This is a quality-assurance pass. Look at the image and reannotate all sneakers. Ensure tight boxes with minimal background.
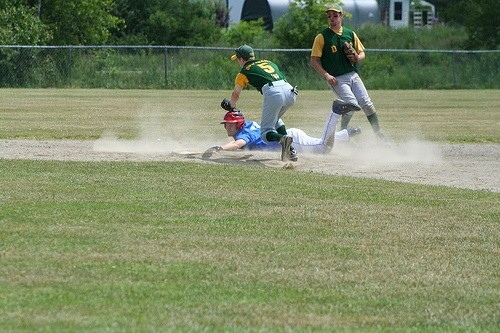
[347,126,361,137]
[278,135,298,162]
[331,99,361,114]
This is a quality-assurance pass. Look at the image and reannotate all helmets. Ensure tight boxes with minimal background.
[220,111,245,128]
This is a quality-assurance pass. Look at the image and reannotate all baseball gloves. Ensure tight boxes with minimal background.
[343,42,359,64]
[220,98,236,112]
[202,145,223,160]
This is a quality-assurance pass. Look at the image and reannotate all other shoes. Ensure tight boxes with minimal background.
[374,132,384,139]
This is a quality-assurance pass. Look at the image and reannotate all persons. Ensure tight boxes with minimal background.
[220,44,299,161]
[202,99,361,159]
[309,2,385,142]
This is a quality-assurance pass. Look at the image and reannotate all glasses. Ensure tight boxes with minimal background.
[327,15,340,18]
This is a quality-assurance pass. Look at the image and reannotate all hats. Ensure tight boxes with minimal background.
[231,44,254,61]
[324,3,343,14]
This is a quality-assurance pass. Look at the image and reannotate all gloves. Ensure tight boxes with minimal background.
[221,98,236,111]
[202,146,223,160]
